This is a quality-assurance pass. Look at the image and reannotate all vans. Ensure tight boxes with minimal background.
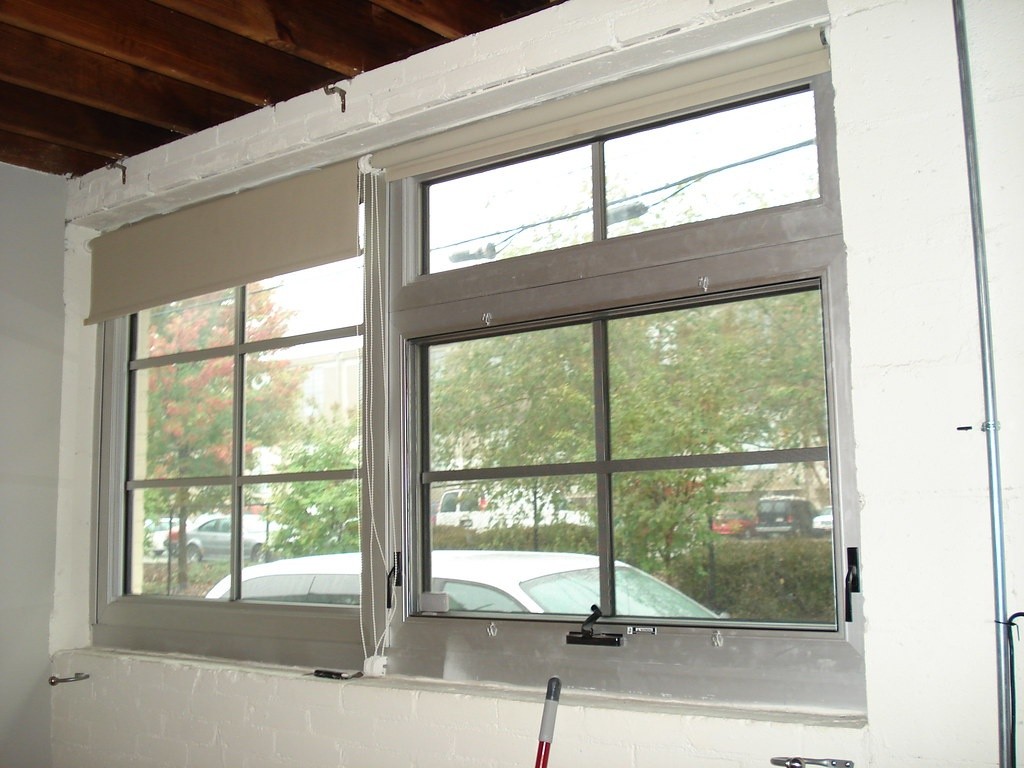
[435,489,550,530]
[205,549,728,618]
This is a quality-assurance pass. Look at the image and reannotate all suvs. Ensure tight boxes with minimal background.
[753,495,819,538]
[145,517,191,557]
[813,506,834,537]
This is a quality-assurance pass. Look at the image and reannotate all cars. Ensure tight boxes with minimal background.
[165,515,303,562]
[713,512,754,538]
